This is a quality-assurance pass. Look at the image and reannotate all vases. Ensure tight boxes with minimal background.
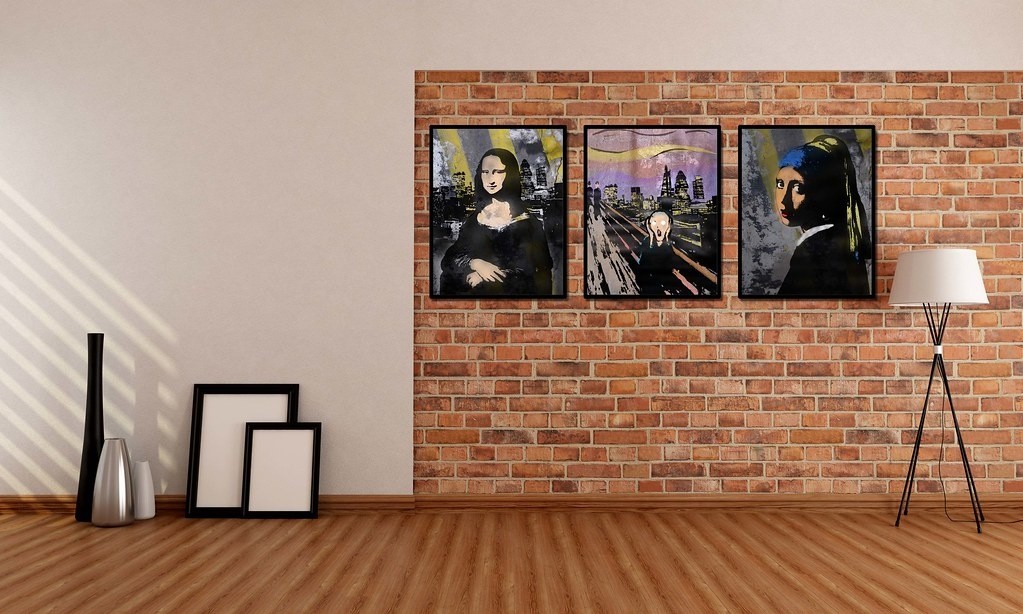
[134,460,155,519]
[92,438,134,527]
[75,333,111,522]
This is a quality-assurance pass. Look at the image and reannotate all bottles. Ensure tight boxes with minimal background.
[92,438,157,527]
[74,332,108,521]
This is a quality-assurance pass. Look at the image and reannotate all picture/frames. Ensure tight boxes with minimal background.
[241,422,322,518]
[185,384,299,518]
[738,125,875,299]
[428,125,567,299]
[584,125,725,300]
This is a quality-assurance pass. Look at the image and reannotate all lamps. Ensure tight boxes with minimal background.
[888,248,990,533]
